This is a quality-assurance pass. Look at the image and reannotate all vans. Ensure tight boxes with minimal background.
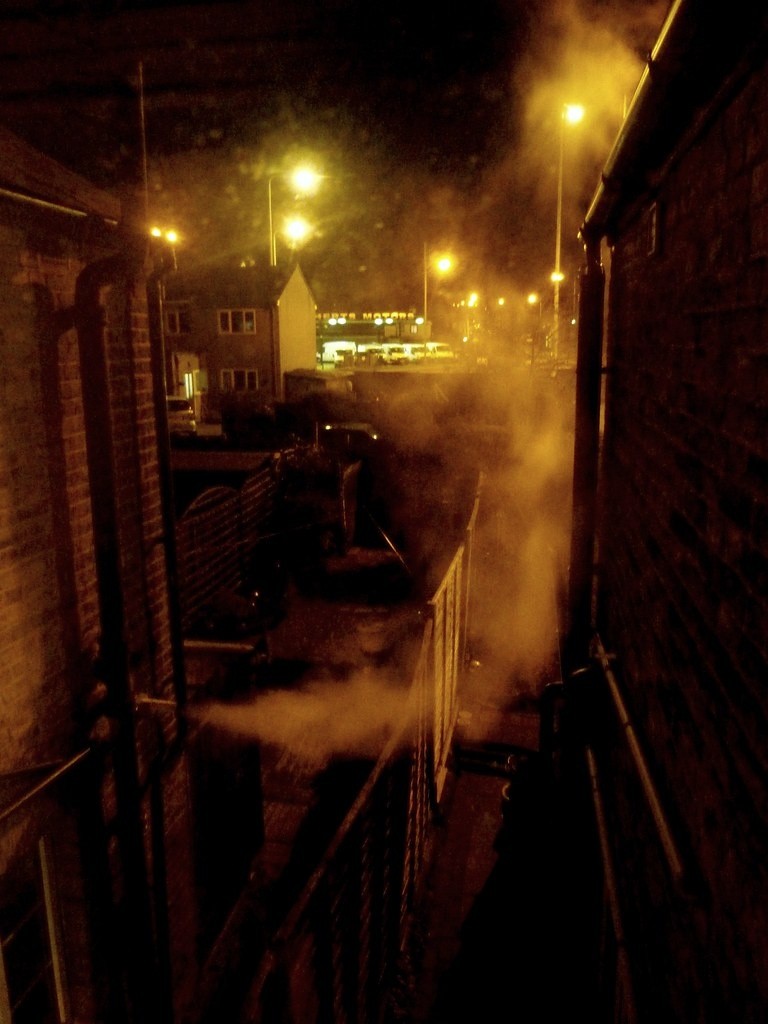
[332,342,455,369]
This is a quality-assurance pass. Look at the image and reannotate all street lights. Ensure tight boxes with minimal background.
[268,164,319,267]
[549,100,587,380]
